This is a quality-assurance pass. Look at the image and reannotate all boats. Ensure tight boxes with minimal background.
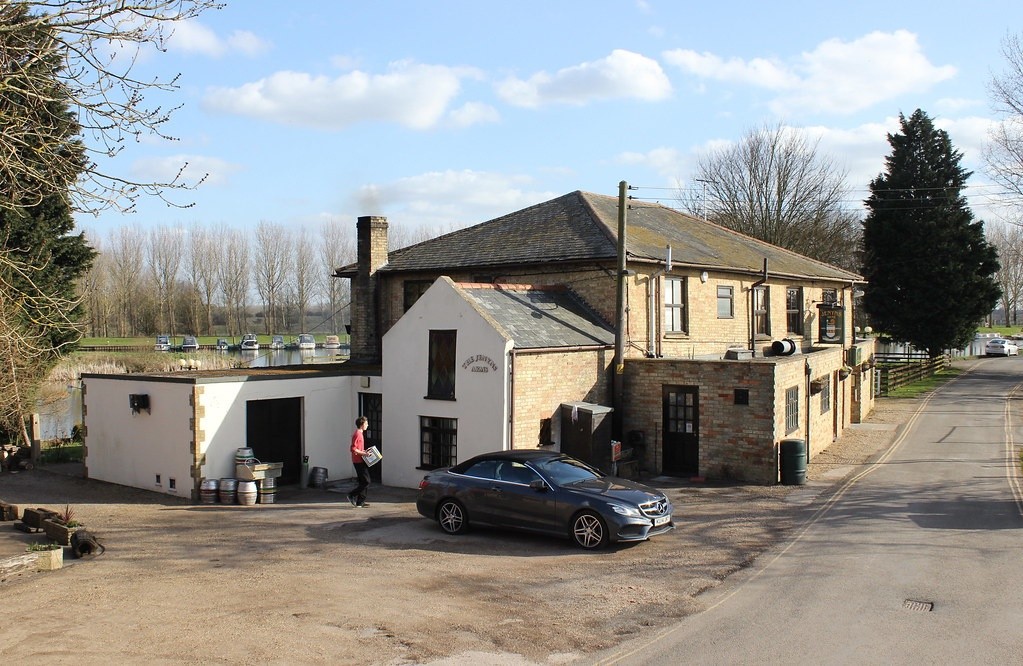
[240,334,260,351]
[296,335,316,349]
[181,337,199,351]
[154,334,171,352]
[323,335,341,349]
[269,336,286,350]
[217,337,229,350]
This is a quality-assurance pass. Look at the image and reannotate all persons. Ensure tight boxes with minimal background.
[347,417,370,508]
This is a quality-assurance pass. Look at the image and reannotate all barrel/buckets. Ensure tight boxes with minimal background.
[237,481,257,505]
[779,439,806,485]
[235,447,255,464]
[219,478,238,504]
[310,467,328,488]
[259,478,274,503]
[200,479,219,503]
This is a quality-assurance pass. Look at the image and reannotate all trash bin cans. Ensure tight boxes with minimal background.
[781,440,807,485]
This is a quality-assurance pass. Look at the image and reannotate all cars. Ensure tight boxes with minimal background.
[417,448,676,551]
[985,339,1020,356]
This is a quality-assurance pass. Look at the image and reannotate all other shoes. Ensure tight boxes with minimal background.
[356,502,370,508]
[346,494,356,507]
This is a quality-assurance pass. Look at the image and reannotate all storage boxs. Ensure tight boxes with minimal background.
[361,446,382,468]
[610,441,622,461]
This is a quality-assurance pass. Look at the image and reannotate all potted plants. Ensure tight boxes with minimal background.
[812,375,826,393]
[862,359,871,370]
[43,504,86,546]
[839,363,850,380]
